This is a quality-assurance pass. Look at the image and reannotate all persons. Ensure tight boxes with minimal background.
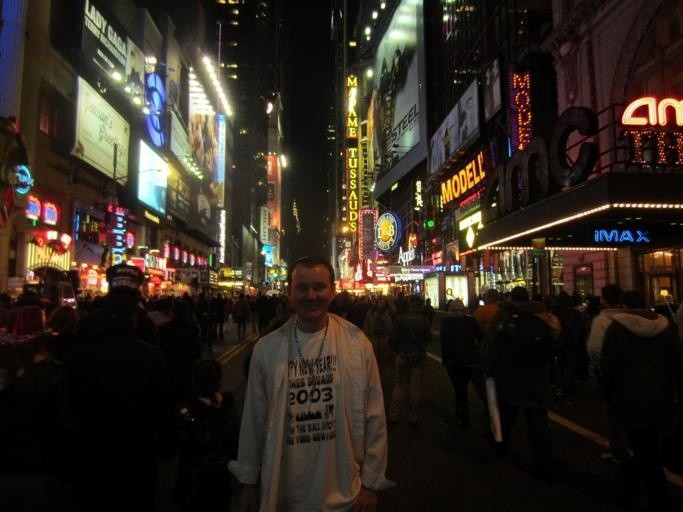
[0,257,683,512]
